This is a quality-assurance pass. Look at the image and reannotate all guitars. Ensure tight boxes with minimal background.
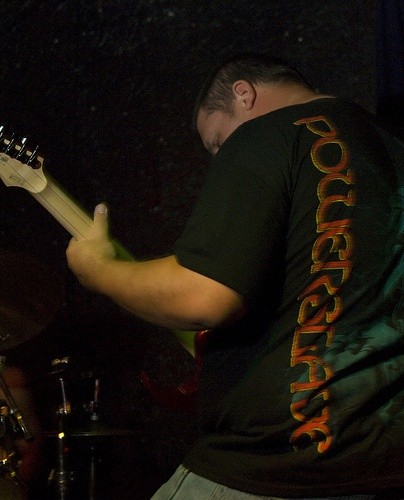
[1,126,256,425]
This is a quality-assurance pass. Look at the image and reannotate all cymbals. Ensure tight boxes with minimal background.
[72,426,147,436]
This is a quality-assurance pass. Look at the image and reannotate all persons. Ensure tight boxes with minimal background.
[64,50,404,500]
